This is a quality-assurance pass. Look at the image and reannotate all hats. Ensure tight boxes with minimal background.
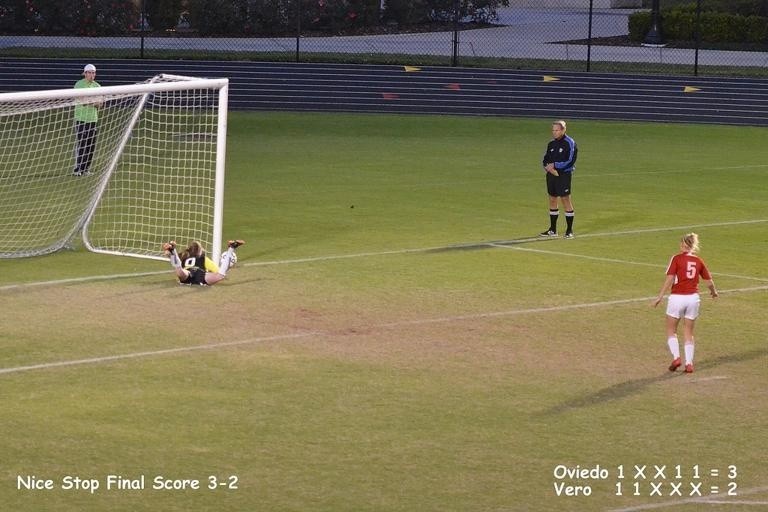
[84,64,96,72]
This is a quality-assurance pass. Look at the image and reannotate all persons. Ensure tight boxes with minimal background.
[649,233,718,373]
[71,63,104,176]
[539,120,577,239]
[162,238,244,286]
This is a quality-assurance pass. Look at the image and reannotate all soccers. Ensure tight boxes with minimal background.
[220,252,236,267]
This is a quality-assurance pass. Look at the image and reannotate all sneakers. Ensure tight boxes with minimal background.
[541,227,575,240]
[163,241,176,250]
[228,240,244,247]
[669,357,694,373]
